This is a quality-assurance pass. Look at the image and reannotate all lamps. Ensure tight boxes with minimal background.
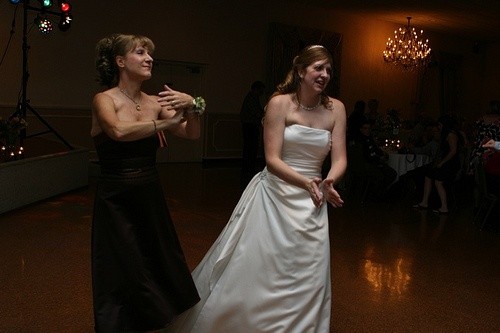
[8,0,73,34]
[383,16,431,73]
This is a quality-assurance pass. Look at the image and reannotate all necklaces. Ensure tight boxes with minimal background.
[295,96,322,111]
[118,87,142,111]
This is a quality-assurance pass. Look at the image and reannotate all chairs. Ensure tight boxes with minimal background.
[471,157,500,231]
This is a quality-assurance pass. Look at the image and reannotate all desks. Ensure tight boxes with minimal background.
[386,154,432,184]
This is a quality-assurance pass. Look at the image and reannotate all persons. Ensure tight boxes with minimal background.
[167,44,347,333]
[325,99,500,214]
[89,34,206,333]
[240,81,264,177]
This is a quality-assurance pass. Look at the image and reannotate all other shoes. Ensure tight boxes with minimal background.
[432,209,448,214]
[412,204,428,208]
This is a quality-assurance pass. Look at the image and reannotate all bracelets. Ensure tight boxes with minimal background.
[192,96,206,119]
[152,120,157,135]
